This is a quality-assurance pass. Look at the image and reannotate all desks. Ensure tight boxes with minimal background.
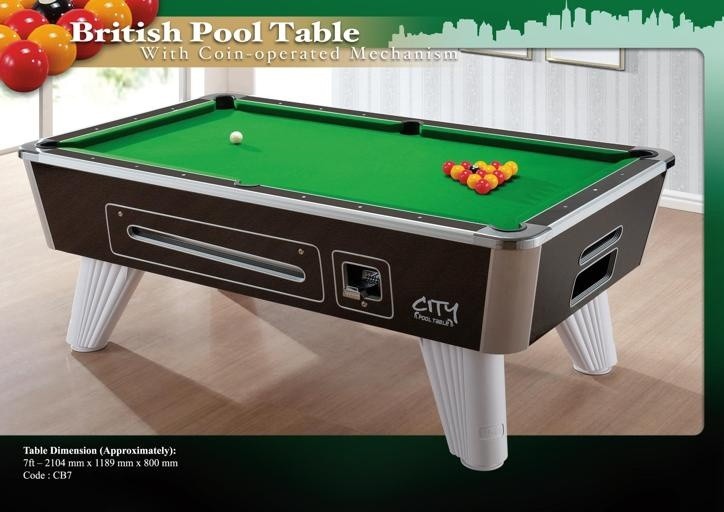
[13,88,677,474]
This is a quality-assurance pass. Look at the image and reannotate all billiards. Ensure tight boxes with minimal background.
[230,130,243,144]
[444,160,518,195]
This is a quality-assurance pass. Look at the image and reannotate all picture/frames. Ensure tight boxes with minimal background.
[543,47,626,73]
[457,47,533,62]
